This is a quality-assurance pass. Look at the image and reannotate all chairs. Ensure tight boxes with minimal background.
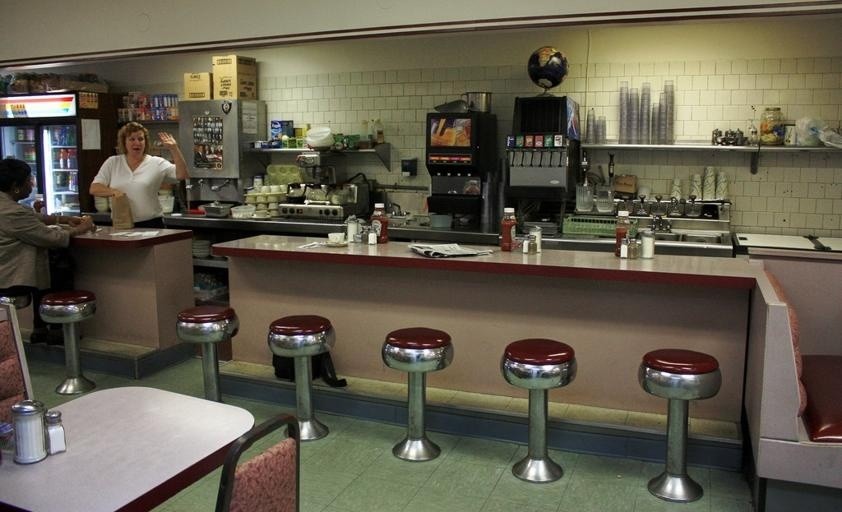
[0,297,43,445]
[214,410,302,511]
[741,265,840,512]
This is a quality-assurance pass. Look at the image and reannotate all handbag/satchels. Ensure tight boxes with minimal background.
[272,353,346,387]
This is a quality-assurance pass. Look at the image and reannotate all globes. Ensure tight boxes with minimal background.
[526,46,571,98]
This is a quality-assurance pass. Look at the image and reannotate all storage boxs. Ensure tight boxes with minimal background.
[183,71,215,103]
[269,119,294,148]
[210,51,260,103]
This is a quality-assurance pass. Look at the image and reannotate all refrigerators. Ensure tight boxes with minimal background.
[1,89,125,215]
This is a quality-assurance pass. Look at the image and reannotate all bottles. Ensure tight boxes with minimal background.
[523,254,541,265]
[254,176,263,191]
[50,126,78,208]
[711,128,743,147]
[348,202,388,245]
[759,106,785,145]
[783,123,796,146]
[639,229,655,259]
[748,127,757,144]
[522,226,542,254]
[614,210,638,259]
[366,118,384,149]
[500,206,517,252]
[12,398,67,465]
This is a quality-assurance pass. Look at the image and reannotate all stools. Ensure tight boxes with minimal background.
[379,325,457,462]
[2,293,33,357]
[36,289,103,398]
[265,314,338,443]
[631,344,723,504]
[496,336,578,484]
[173,303,243,406]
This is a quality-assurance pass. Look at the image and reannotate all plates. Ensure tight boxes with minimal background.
[326,241,348,247]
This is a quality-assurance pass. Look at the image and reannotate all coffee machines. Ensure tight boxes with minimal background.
[177,99,268,211]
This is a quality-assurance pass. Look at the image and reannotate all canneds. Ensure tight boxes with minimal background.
[17,127,36,142]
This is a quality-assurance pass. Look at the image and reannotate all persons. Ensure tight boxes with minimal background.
[89,121,190,228]
[0,159,93,349]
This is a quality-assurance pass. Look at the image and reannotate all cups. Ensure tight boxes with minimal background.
[327,232,346,242]
[669,177,682,199]
[689,164,730,202]
[575,182,594,211]
[461,91,492,113]
[244,184,287,218]
[594,183,614,213]
[158,195,175,214]
[618,78,675,146]
[585,109,606,145]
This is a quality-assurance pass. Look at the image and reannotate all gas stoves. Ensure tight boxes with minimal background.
[278,202,371,220]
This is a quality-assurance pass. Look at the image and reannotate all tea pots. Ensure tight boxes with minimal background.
[285,181,354,205]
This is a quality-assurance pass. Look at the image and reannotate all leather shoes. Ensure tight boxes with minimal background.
[31,333,63,345]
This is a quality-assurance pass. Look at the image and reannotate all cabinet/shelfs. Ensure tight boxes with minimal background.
[115,119,180,152]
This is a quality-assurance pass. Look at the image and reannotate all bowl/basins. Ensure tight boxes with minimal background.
[231,204,256,217]
[93,194,112,213]
[432,213,452,228]
[192,238,226,260]
